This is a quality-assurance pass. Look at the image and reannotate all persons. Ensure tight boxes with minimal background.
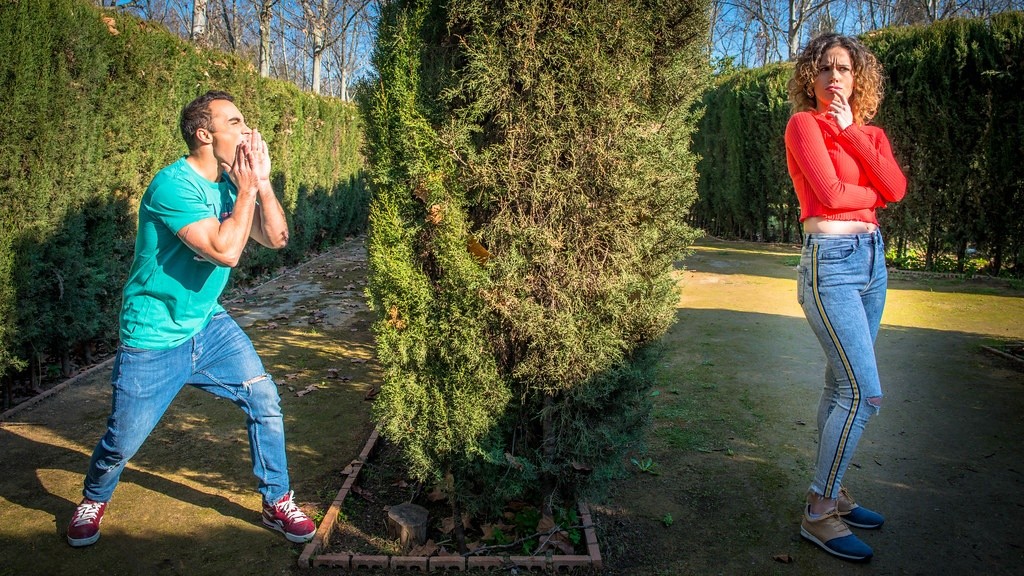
[785,34,907,559]
[67,90,316,546]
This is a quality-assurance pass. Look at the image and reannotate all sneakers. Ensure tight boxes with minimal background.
[805,486,885,529]
[799,503,872,559]
[262,491,317,542]
[67,498,107,546]
